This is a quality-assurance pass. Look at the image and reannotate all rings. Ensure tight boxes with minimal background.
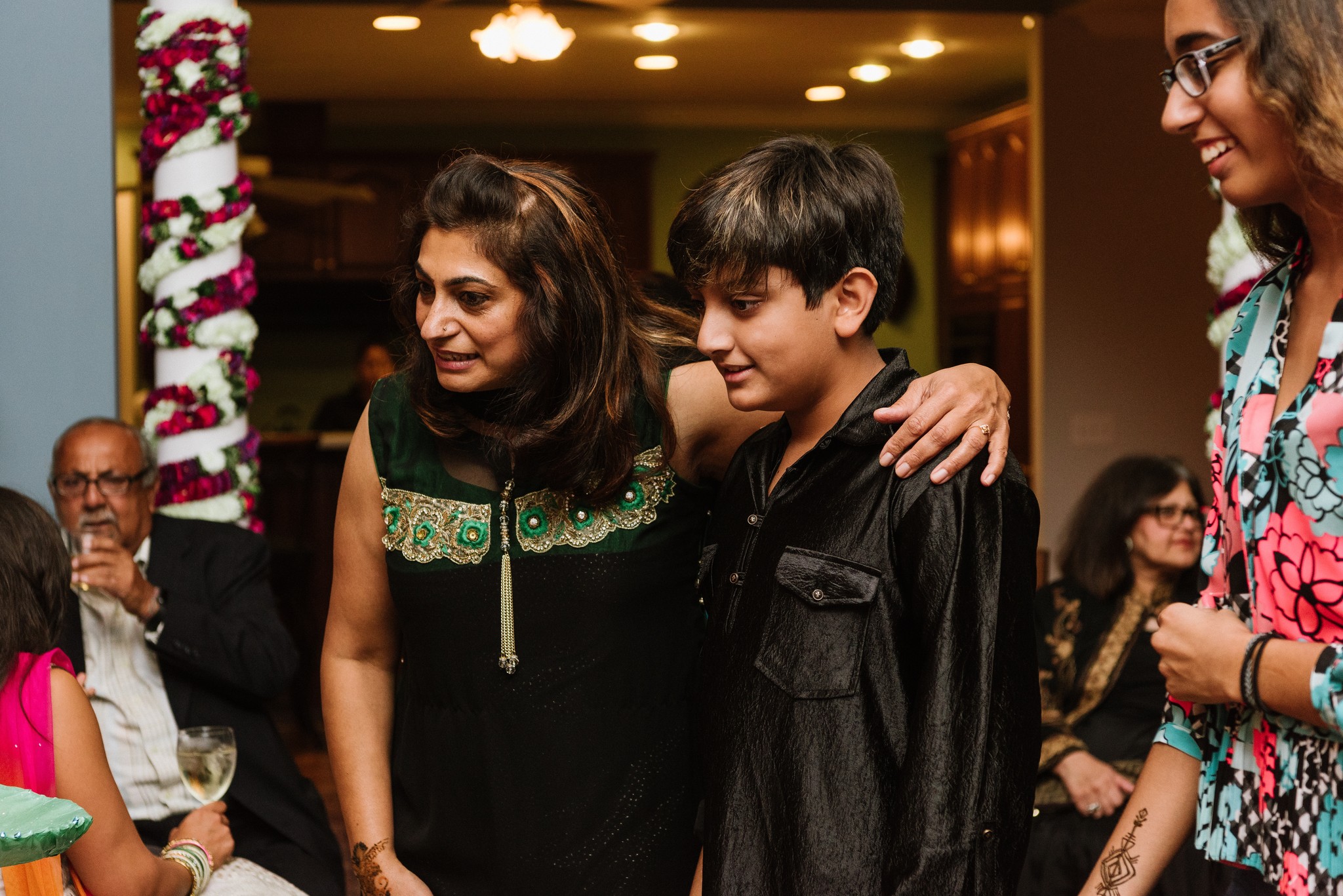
[969,424,990,440]
[1087,803,1099,815]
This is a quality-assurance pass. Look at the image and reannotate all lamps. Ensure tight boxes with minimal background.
[470,0,580,63]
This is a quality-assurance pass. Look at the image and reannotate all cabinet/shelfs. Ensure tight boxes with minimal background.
[942,99,1027,487]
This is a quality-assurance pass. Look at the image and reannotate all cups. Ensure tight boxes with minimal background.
[176,726,237,808]
[69,531,119,598]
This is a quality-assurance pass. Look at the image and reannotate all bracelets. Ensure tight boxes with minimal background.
[159,838,214,896]
[1236,631,1285,714]
[145,588,167,633]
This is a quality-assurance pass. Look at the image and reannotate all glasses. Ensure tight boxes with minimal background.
[1143,504,1206,527]
[48,465,151,498]
[1158,34,1244,98]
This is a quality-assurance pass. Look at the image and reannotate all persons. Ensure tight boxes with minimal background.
[322,150,1012,896]
[665,135,1043,894]
[0,485,235,896]
[46,416,346,895]
[309,338,403,432]
[1035,453,1206,896]
[1074,0,1343,896]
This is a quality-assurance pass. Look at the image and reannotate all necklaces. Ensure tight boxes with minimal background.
[488,455,524,674]
